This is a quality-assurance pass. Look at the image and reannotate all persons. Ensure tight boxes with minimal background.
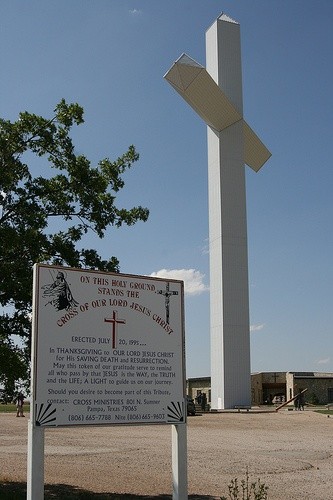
[200,392,207,412]
[297,392,305,410]
[14,392,26,417]
